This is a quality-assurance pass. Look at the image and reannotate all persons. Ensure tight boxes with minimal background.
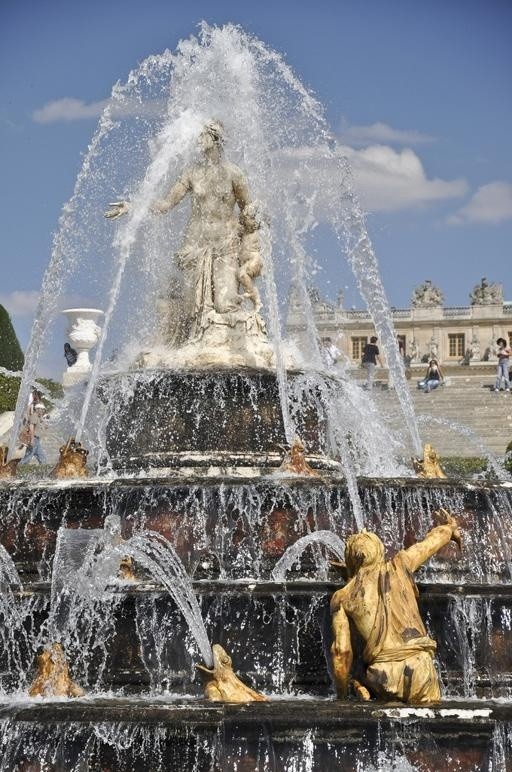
[494,337,512,392]
[17,402,48,467]
[414,279,442,305]
[359,335,385,389]
[418,359,444,392]
[474,277,496,305]
[327,507,465,702]
[237,211,267,314]
[104,118,259,317]
[62,341,77,366]
[426,336,438,358]
[323,337,342,367]
